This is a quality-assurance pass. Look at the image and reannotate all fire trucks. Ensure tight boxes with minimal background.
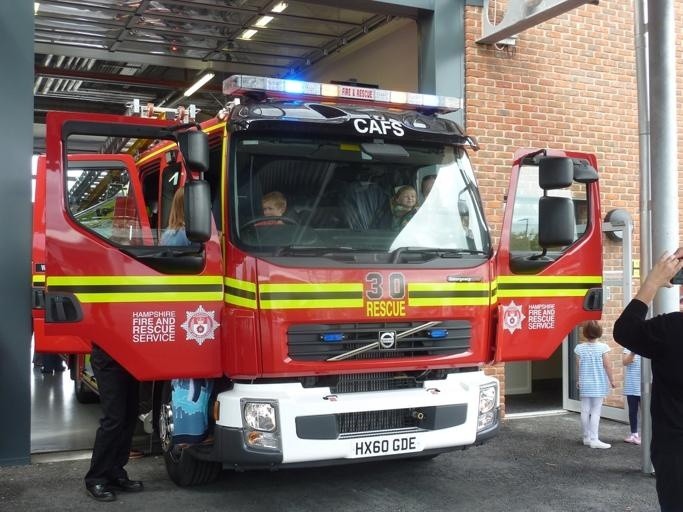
[26,74,607,491]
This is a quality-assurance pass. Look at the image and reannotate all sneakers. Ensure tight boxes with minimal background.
[624,434,635,443]
[590,439,612,450]
[40,366,67,373]
[583,438,591,446]
[633,436,641,446]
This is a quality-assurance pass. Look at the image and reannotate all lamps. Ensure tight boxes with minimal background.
[183,69,216,97]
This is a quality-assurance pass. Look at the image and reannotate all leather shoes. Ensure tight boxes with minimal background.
[86,480,117,502]
[114,477,145,493]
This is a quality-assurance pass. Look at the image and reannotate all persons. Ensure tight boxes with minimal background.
[157,185,213,449]
[40,353,66,373]
[32,350,43,366]
[391,185,418,231]
[254,191,287,226]
[622,347,642,445]
[421,174,438,200]
[82,237,144,503]
[574,320,617,449]
[611,245,683,512]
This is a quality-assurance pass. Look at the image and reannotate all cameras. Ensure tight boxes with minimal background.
[670,267,683,284]
